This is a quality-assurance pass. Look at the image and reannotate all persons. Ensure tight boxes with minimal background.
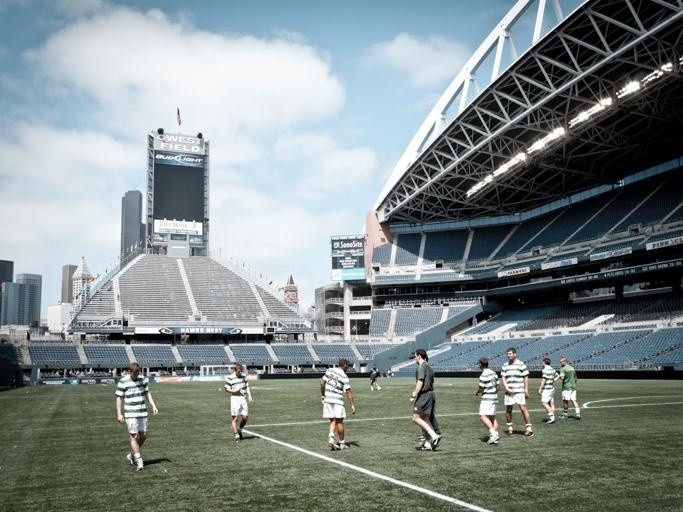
[370,364,382,391]
[538,358,560,424]
[559,358,581,419]
[321,358,356,451]
[387,368,392,378]
[224,364,253,441]
[68,368,94,377]
[409,348,443,451]
[476,358,501,444]
[115,363,158,472]
[501,348,534,436]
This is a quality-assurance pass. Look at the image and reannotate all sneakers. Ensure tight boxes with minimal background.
[370,387,382,391]
[486,433,501,445]
[523,430,534,438]
[431,434,443,451]
[546,412,581,425]
[327,438,349,451]
[133,456,143,472]
[503,428,513,438]
[126,452,135,466]
[233,429,244,441]
[416,440,433,450]
